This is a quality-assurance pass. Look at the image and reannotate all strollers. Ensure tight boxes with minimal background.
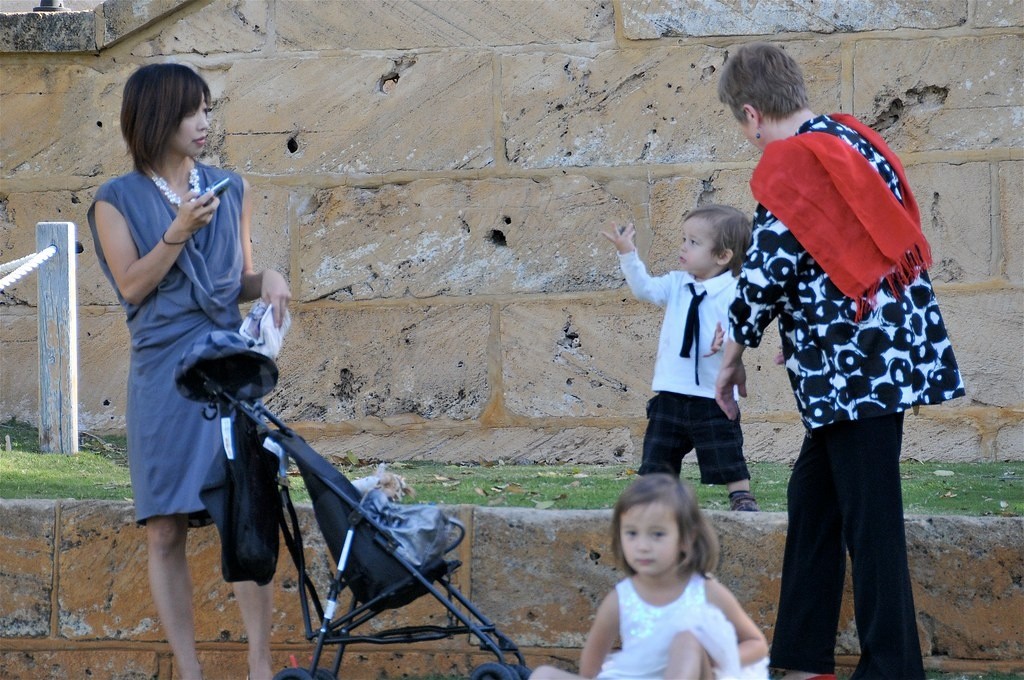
[200,368,532,680]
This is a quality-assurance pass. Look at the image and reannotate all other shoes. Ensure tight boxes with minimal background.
[731,493,758,511]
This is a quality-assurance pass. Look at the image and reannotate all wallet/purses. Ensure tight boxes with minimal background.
[238,302,292,360]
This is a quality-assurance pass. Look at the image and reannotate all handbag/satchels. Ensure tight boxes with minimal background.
[361,486,447,566]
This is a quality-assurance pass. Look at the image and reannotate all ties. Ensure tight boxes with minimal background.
[679,282,710,387]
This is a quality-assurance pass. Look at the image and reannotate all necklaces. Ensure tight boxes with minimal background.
[151,168,201,208]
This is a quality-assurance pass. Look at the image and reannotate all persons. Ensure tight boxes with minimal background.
[526,473,770,680]
[715,44,966,680]
[86,63,292,680]
[601,205,759,512]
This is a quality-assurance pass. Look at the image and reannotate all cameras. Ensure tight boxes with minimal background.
[196,177,230,208]
